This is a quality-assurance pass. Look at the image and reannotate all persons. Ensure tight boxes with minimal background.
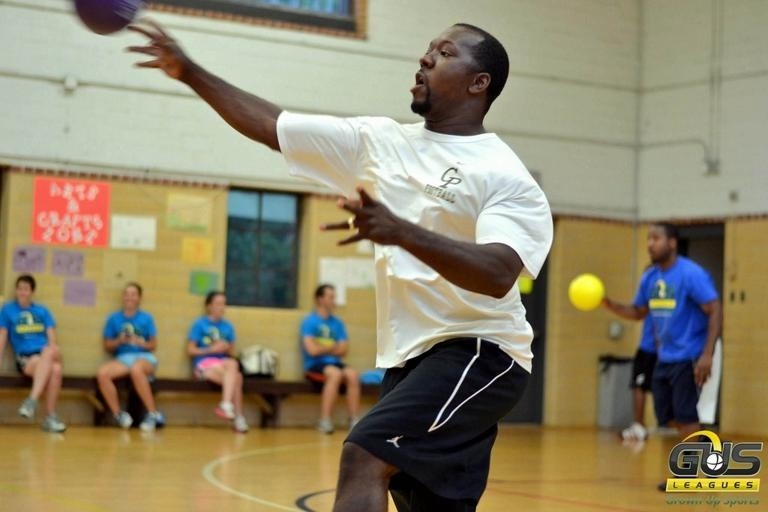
[95,281,168,433]
[185,291,251,433]
[123,16,557,512]
[0,274,68,433]
[298,283,363,434]
[617,316,658,440]
[602,223,724,493]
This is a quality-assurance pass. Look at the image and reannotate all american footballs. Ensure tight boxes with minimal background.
[75,1,136,34]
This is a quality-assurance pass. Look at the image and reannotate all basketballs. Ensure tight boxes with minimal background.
[569,273,604,311]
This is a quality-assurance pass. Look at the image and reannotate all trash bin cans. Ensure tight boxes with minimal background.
[597,354,634,428]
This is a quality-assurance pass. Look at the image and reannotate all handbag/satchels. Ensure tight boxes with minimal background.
[242,344,275,379]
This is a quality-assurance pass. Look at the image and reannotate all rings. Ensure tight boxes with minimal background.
[347,218,356,230]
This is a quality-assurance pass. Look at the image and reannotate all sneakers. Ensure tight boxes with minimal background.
[18,398,66,432]
[115,411,165,430]
[620,422,647,439]
[215,401,248,432]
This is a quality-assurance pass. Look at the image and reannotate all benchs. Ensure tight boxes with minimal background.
[0,377,378,429]
